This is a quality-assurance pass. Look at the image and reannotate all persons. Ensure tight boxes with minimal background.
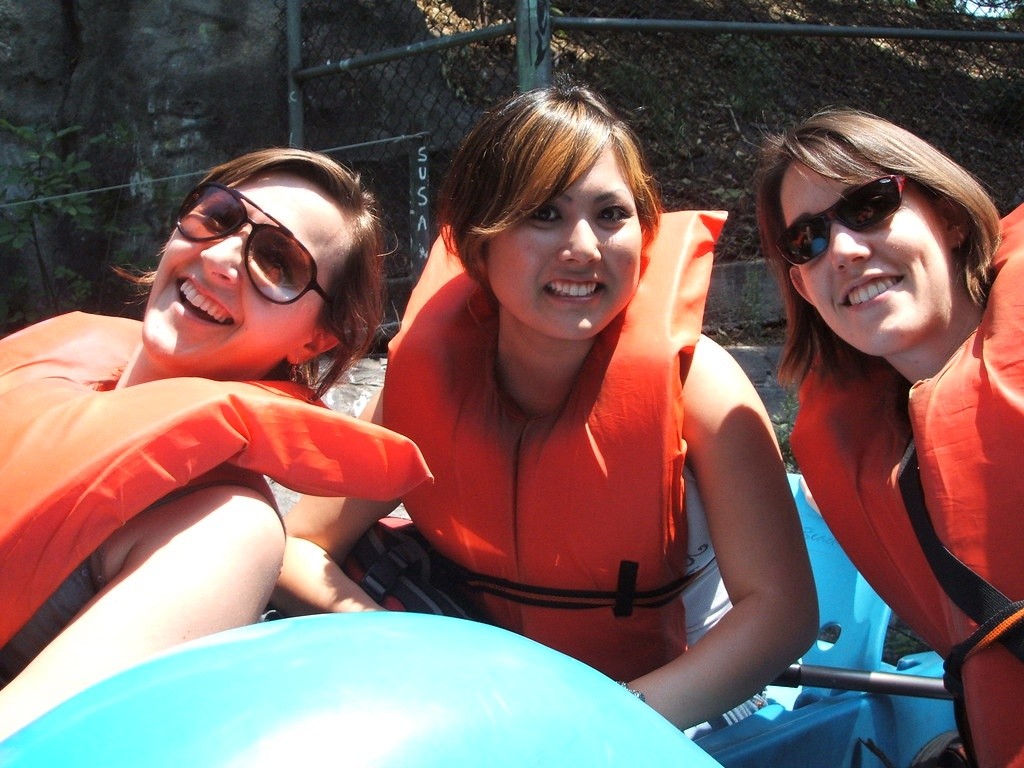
[275,83,820,731]
[755,108,1024,768]
[0,146,436,743]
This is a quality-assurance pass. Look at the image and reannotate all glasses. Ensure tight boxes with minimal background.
[775,175,908,266]
[176,180,338,312]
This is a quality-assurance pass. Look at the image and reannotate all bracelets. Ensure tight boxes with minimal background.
[619,680,646,704]
